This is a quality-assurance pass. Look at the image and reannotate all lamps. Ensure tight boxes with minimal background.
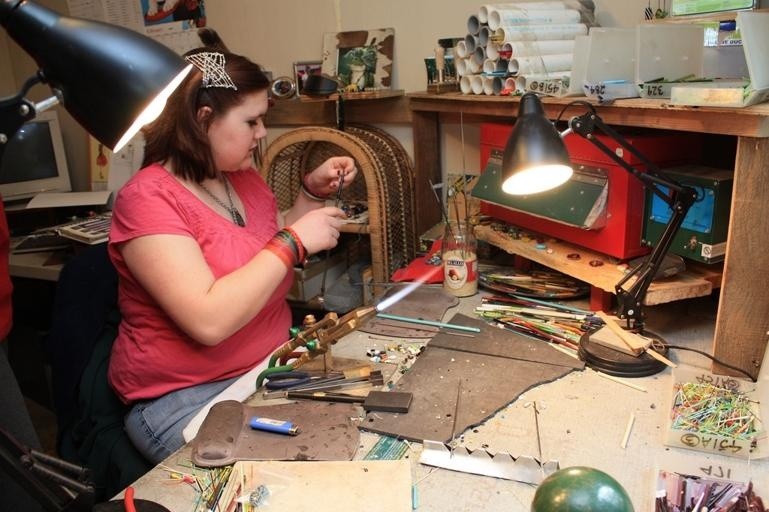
[502,90,701,379]
[1,1,190,154]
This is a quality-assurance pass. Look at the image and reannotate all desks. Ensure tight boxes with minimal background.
[119,263,769,512]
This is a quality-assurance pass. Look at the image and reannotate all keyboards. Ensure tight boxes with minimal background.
[12,234,81,254]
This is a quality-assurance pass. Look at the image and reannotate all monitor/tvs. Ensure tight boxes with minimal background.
[0,109,73,201]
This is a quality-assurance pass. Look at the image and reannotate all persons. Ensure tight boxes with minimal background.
[107,49,359,470]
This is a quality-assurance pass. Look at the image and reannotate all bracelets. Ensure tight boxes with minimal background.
[301,172,331,204]
[263,227,308,270]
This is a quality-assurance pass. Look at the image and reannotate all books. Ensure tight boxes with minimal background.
[61,208,116,246]
[12,226,72,256]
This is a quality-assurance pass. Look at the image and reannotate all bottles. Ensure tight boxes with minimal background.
[441,222,479,298]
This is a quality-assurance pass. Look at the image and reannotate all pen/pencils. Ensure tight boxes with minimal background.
[654,481,742,512]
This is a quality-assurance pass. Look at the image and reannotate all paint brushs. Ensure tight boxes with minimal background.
[473,268,607,351]
